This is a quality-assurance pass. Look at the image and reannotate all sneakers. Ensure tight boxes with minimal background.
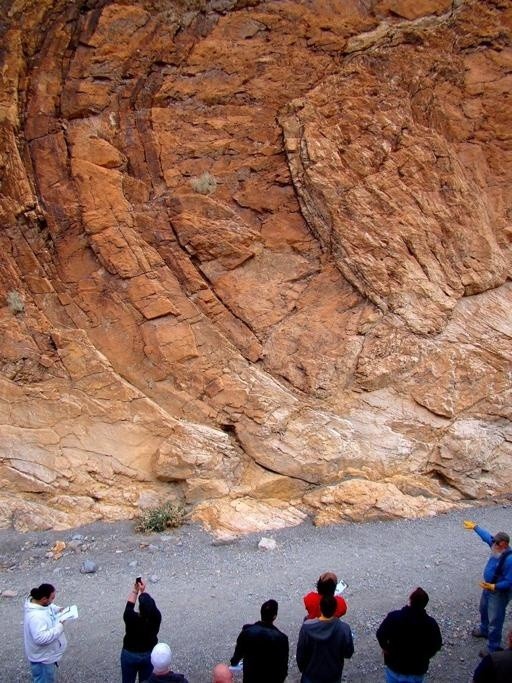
[479,649,488,657]
[473,629,481,636]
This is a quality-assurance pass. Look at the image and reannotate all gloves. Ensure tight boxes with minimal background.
[480,582,496,592]
[463,521,476,529]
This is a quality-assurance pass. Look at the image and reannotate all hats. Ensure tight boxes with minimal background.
[139,593,155,611]
[409,587,429,604]
[151,642,172,672]
[491,532,510,542]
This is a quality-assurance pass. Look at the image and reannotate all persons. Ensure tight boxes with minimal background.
[296,595,354,683]
[120,576,161,683]
[472,627,511,683]
[229,599,289,683]
[375,587,441,683]
[23,583,66,683]
[463,519,512,658]
[303,572,347,621]
[142,643,189,683]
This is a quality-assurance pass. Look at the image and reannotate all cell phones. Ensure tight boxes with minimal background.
[135,576,142,585]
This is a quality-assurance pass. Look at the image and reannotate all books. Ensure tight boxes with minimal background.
[55,605,78,624]
[334,579,349,596]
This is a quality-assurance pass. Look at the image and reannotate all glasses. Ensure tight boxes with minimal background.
[491,539,499,545]
